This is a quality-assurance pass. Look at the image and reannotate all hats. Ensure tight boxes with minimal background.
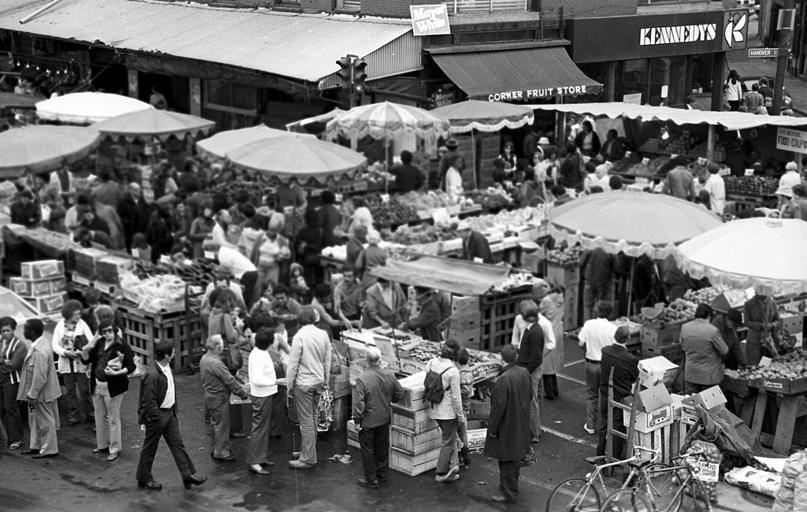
[365,230,381,243]
[726,308,743,325]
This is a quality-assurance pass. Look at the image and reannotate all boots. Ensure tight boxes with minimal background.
[542,395,554,400]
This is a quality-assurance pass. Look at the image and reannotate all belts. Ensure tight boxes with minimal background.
[584,357,601,364]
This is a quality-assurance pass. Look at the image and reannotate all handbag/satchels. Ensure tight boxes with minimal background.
[220,313,244,371]
[518,443,538,466]
[107,352,125,370]
[314,386,334,433]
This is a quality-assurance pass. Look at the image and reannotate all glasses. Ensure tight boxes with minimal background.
[100,329,113,335]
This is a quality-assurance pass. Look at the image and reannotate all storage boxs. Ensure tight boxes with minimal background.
[230,385,252,404]
[638,356,679,388]
[347,419,363,449]
[637,382,673,413]
[389,407,443,477]
[639,326,679,346]
[466,420,488,449]
[329,397,349,431]
[673,384,728,426]
[22,296,71,317]
[711,288,745,309]
[623,403,673,433]
[776,310,803,348]
[391,370,430,412]
[21,260,66,282]
[9,277,68,298]
[444,295,481,350]
[330,383,352,399]
[97,256,132,284]
[73,248,108,276]
[691,454,722,482]
[468,397,491,420]
[776,299,807,317]
[641,343,681,362]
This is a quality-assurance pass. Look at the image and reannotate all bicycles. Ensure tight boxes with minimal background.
[545,445,684,512]
[600,451,712,512]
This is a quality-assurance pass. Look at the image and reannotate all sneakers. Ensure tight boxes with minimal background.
[224,454,235,460]
[289,460,314,468]
[449,463,459,473]
[434,473,459,481]
[233,432,246,437]
[8,439,25,450]
[583,422,595,434]
[293,452,300,457]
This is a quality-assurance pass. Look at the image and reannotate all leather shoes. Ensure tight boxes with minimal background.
[31,453,58,459]
[260,460,274,467]
[357,477,379,488]
[137,479,162,491]
[21,448,39,455]
[182,474,207,489]
[375,473,388,481]
[67,421,74,427]
[92,447,108,453]
[106,452,119,461]
[491,495,507,503]
[91,425,96,432]
[247,464,271,475]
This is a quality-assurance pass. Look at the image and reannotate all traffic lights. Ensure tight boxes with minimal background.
[336,54,367,109]
